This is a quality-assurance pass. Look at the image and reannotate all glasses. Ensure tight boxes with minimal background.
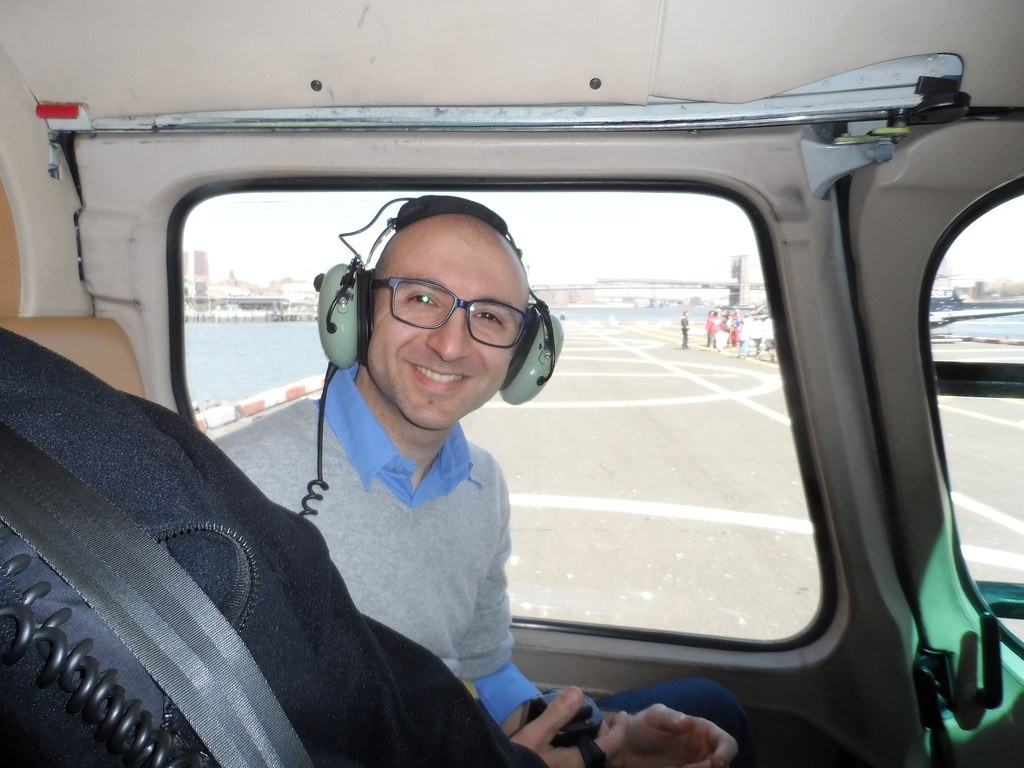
[370,277,536,349]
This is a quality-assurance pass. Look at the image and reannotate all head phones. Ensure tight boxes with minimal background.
[313,195,564,405]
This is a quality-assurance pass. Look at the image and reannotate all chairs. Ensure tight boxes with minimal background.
[1,181,148,404]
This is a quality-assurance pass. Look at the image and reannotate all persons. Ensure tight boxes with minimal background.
[208,212,740,768]
[679,306,778,363]
